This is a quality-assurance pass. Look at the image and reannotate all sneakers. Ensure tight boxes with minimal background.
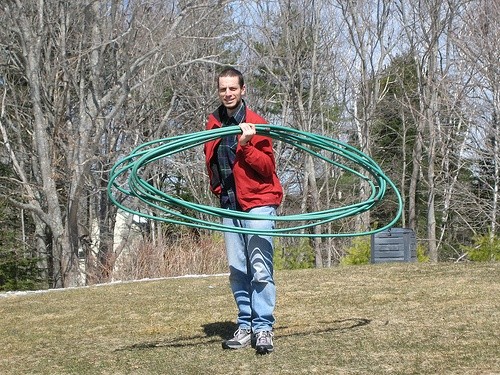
[255,331,274,354]
[222,326,254,349]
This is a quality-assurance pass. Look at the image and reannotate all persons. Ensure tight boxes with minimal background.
[203,68,284,353]
